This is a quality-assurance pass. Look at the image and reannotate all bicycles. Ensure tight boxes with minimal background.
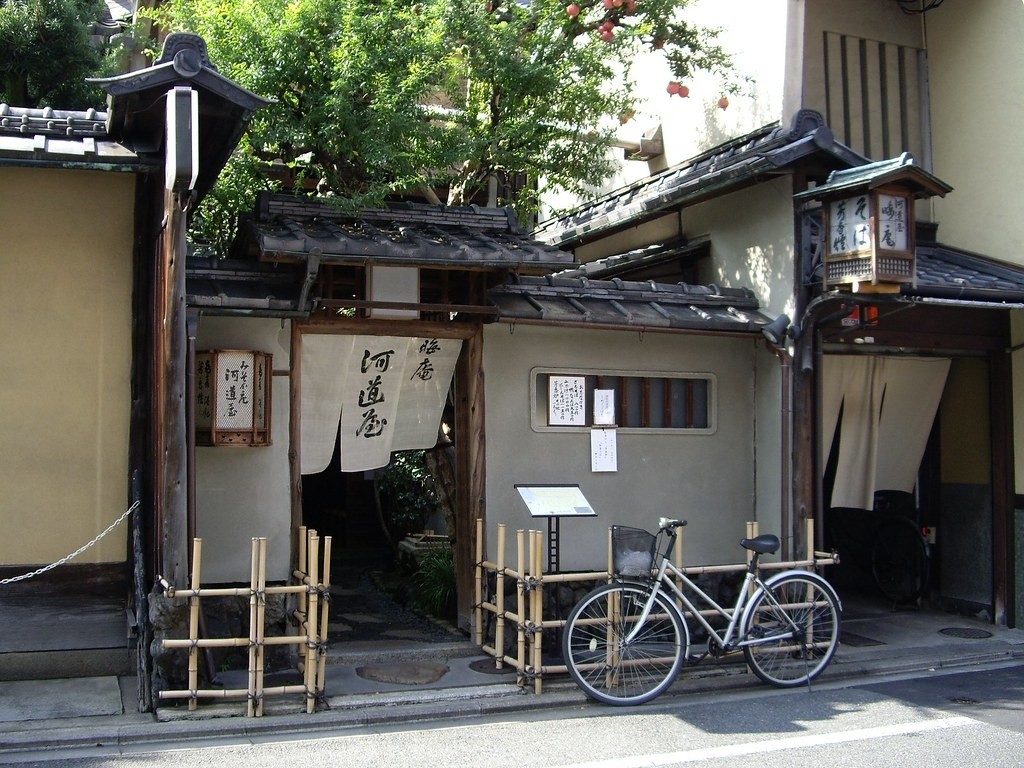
[561,515,847,707]
[825,492,932,607]
